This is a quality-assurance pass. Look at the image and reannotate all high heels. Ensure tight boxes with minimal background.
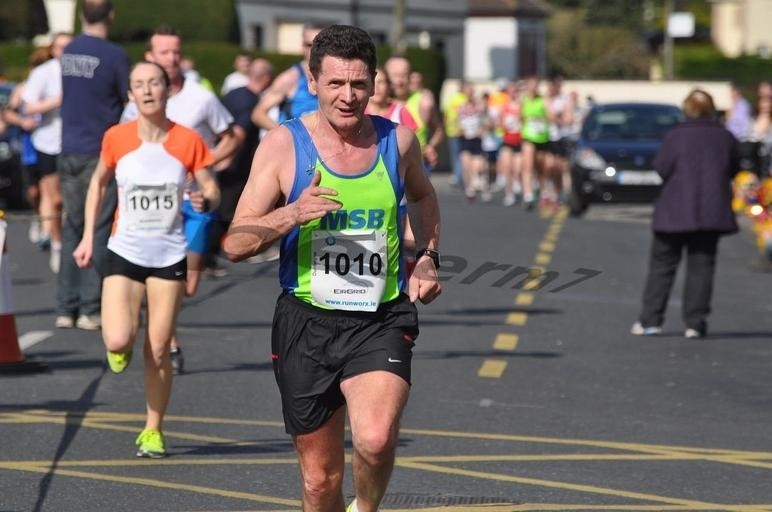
[0,223,28,369]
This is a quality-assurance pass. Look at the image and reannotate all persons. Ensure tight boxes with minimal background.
[629,89,740,339]
[219,49,253,94]
[748,81,772,178]
[440,69,592,218]
[74,61,222,458]
[253,23,332,132]
[119,27,244,372]
[223,25,441,512]
[724,80,755,173]
[179,56,216,94]
[365,55,445,301]
[1,33,74,274]
[55,0,130,329]
[210,57,279,276]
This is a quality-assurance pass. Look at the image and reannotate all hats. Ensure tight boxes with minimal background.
[1,77,39,215]
[555,104,686,218]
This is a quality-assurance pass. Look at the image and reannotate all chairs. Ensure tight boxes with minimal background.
[105,348,129,375]
[685,327,699,339]
[55,314,100,330]
[631,321,663,336]
[135,427,167,459]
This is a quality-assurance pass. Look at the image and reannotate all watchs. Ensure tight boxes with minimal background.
[416,248,441,271]
